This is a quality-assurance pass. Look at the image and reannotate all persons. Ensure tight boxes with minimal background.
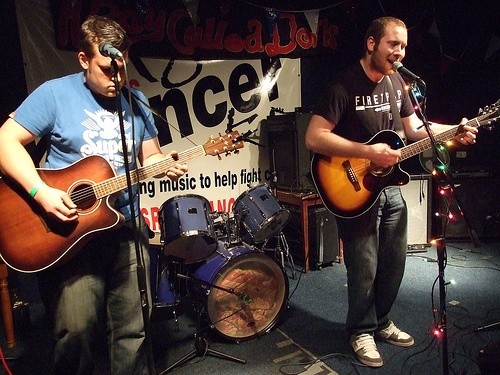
[304,16,478,367]
[0,15,189,375]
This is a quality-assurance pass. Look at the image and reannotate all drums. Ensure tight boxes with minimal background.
[173,239,290,345]
[139,232,180,308]
[231,183,290,245]
[156,193,220,264]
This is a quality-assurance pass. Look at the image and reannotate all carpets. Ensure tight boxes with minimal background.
[273,240,500,375]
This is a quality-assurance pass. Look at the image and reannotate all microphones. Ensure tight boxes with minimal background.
[98,42,123,57]
[242,291,253,305]
[486,216,494,225]
[391,61,426,86]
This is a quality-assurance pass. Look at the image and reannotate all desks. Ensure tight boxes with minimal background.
[273,190,343,274]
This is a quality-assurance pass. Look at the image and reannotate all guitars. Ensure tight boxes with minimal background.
[0,130,245,274]
[311,99,500,219]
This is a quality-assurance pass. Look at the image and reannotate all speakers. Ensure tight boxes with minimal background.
[401,173,433,252]
[306,208,339,268]
[263,114,317,194]
[440,172,500,239]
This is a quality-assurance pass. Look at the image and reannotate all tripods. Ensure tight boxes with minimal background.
[260,150,297,280]
[159,274,248,375]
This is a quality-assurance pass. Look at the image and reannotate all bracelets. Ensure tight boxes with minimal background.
[29,181,47,198]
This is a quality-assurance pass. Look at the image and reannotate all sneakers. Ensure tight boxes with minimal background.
[349,333,383,367]
[376,321,414,346]
